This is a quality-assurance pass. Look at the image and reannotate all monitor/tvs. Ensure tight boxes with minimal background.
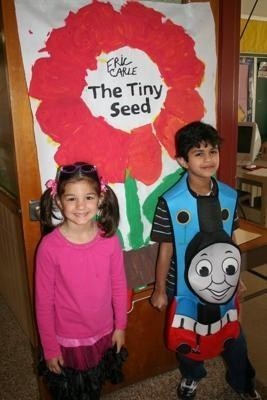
[236,122,262,172]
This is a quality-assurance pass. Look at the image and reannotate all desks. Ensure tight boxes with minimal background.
[236,161,267,230]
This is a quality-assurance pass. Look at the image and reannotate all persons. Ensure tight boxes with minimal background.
[149,119,265,400]
[34,161,130,399]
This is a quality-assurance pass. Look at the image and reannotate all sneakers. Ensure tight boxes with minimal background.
[177,376,201,400]
[239,389,262,400]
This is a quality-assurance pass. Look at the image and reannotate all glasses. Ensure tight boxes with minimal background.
[55,160,98,184]
